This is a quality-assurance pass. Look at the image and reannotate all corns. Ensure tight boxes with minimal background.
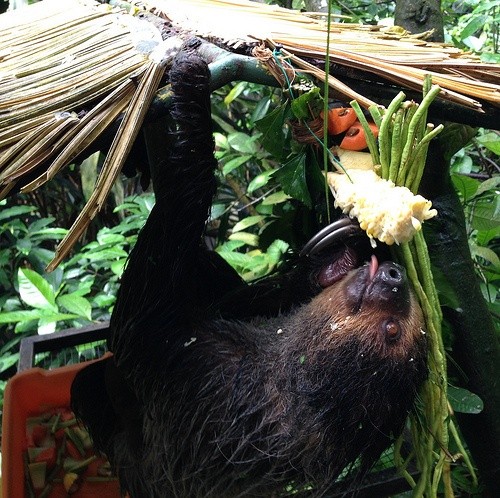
[328,167,439,248]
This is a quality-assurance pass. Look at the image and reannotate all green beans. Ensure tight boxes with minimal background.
[350,75,479,498]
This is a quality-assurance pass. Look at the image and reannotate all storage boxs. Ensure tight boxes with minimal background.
[0,352,132,498]
